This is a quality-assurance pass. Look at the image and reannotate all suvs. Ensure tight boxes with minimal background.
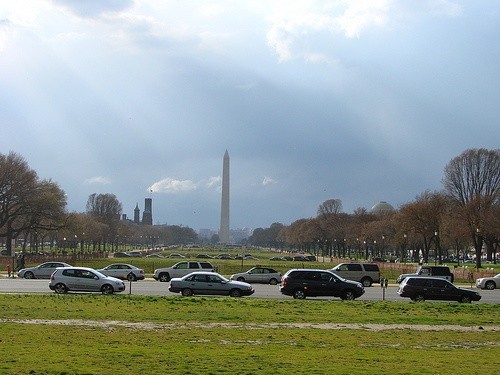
[230,265,282,285]
[279,269,365,300]
[153,261,215,282]
[396,265,454,284]
[396,277,481,303]
[326,263,381,287]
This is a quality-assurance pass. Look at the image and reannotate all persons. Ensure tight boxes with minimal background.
[14,254,26,272]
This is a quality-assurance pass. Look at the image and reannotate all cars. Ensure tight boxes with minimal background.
[168,271,255,297]
[49,267,125,294]
[476,274,500,290]
[270,255,316,261]
[18,262,74,279]
[96,263,145,282]
[114,251,185,258]
[196,253,259,260]
